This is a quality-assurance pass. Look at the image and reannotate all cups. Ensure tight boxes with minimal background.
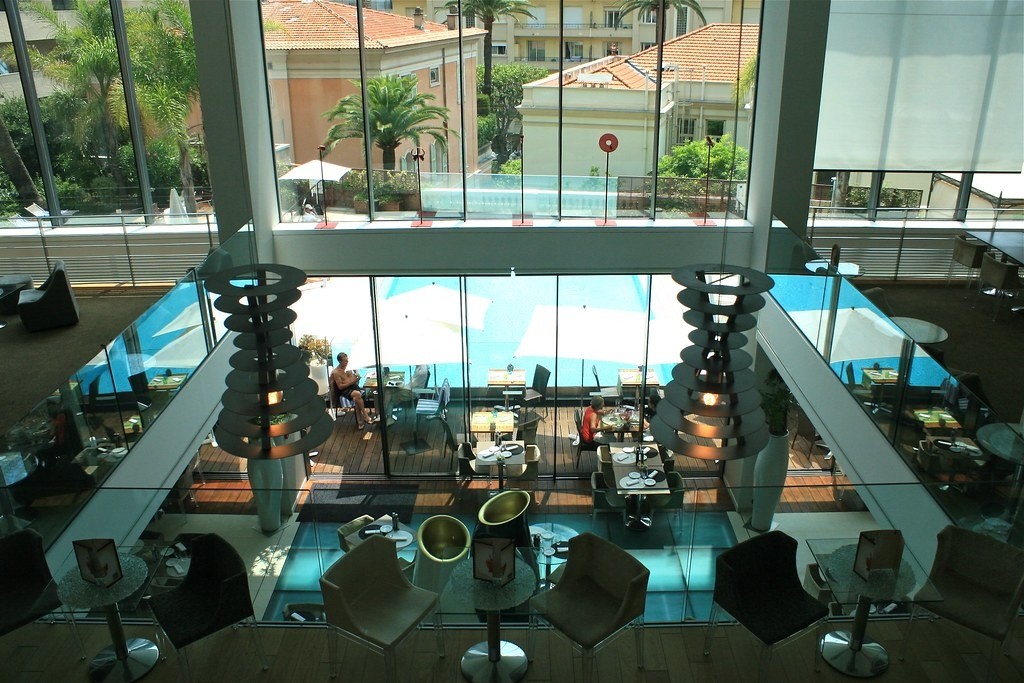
[885,371,889,378]
[493,410,497,418]
[961,448,965,455]
[384,367,389,376]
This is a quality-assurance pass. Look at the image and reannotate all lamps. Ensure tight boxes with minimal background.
[651,0,770,461]
[204,0,333,457]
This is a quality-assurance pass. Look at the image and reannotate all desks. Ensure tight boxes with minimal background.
[167,558,191,577]
[488,369,526,419]
[530,523,580,591]
[926,435,983,494]
[48,378,83,412]
[805,257,866,278]
[966,231,1024,296]
[344,514,419,553]
[612,466,671,529]
[125,415,144,438]
[475,440,525,498]
[0,283,26,328]
[806,537,944,677]
[976,423,1024,549]
[34,545,170,683]
[148,373,187,398]
[914,408,963,431]
[0,451,40,538]
[471,411,515,441]
[862,367,901,415]
[600,412,651,441]
[364,371,406,423]
[70,446,125,468]
[873,316,949,422]
[609,442,663,468]
[433,545,548,683]
[619,368,660,412]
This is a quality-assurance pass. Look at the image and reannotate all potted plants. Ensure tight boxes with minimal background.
[353,192,404,214]
[245,413,293,534]
[749,377,801,531]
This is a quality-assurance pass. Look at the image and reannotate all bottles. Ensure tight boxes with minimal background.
[392,512,398,531]
[495,431,499,446]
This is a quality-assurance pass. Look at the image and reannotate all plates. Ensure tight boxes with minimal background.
[165,558,179,567]
[489,446,499,452]
[501,451,512,457]
[623,446,634,453]
[541,530,554,540]
[629,472,641,479]
[869,604,876,613]
[948,445,962,452]
[644,479,656,486]
[161,548,174,556]
[379,524,392,533]
[543,546,555,556]
[111,447,125,453]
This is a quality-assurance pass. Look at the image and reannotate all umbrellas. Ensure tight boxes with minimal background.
[278,159,352,205]
[143,280,696,410]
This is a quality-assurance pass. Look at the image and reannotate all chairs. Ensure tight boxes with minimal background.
[0,235,1024,683]
[8,203,80,227]
[194,192,213,210]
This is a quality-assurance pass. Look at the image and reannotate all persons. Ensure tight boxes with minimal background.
[386,364,430,427]
[332,352,374,429]
[582,396,617,444]
[639,394,661,430]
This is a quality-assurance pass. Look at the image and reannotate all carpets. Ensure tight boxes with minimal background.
[295,483,420,525]
